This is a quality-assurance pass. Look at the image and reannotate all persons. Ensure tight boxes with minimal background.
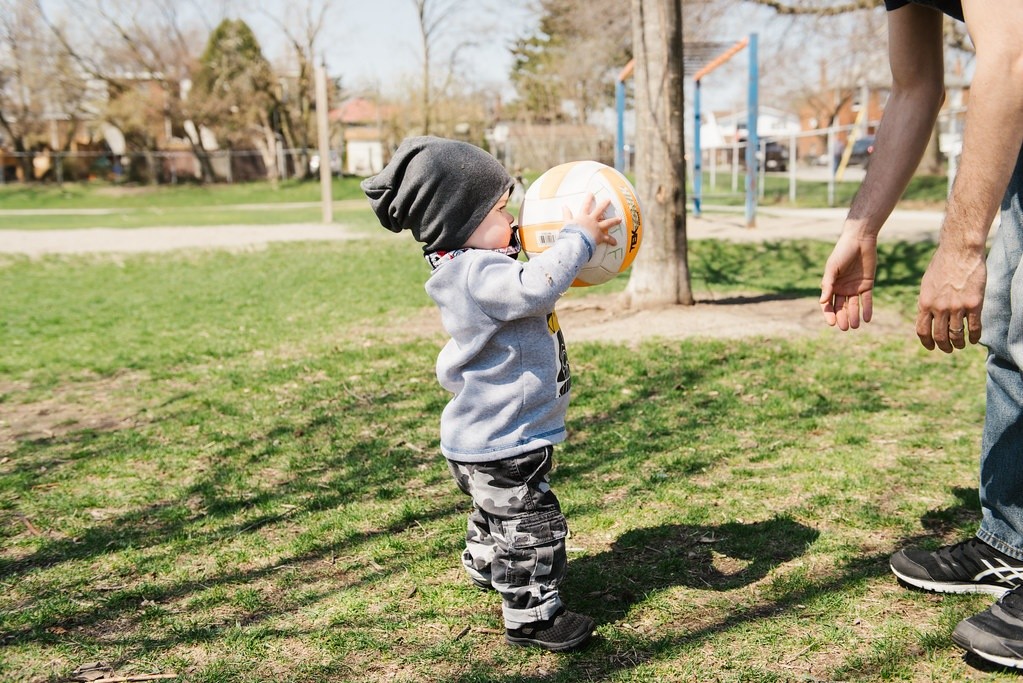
[360,135,623,652]
[818,1,1023,675]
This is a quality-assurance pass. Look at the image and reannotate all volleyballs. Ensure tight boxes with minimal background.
[518,159,644,288]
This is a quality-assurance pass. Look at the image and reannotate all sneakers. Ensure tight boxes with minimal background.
[889,536,1023,596]
[950,582,1023,670]
[505,606,596,651]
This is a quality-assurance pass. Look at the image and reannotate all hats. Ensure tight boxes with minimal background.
[360,135,516,256]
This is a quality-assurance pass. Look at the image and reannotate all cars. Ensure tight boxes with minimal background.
[833,135,877,166]
[738,138,788,172]
